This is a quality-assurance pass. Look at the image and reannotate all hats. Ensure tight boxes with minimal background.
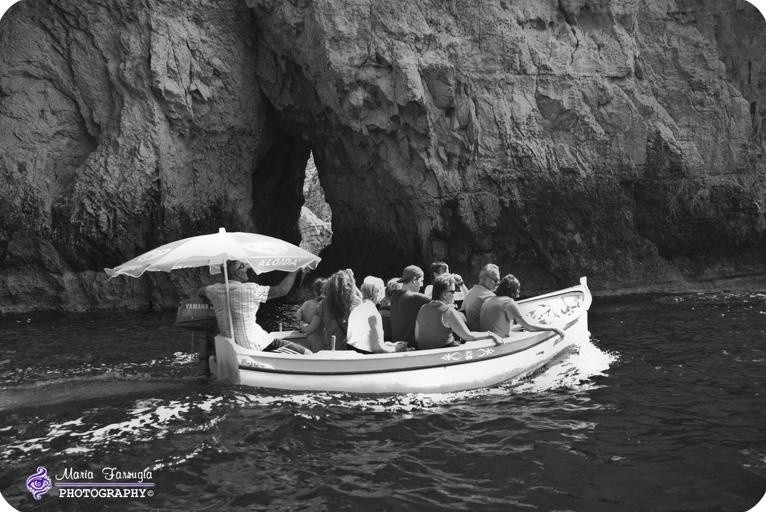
[396,264,424,283]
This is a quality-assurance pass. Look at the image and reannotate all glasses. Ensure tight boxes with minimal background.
[236,263,245,272]
[448,290,455,294]
[486,275,497,283]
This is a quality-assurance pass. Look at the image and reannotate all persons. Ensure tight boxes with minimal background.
[198,259,313,356]
[294,261,565,356]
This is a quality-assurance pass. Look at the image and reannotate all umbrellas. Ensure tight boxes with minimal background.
[103,227,321,344]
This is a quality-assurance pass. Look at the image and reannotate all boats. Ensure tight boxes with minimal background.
[205,274,595,400]
